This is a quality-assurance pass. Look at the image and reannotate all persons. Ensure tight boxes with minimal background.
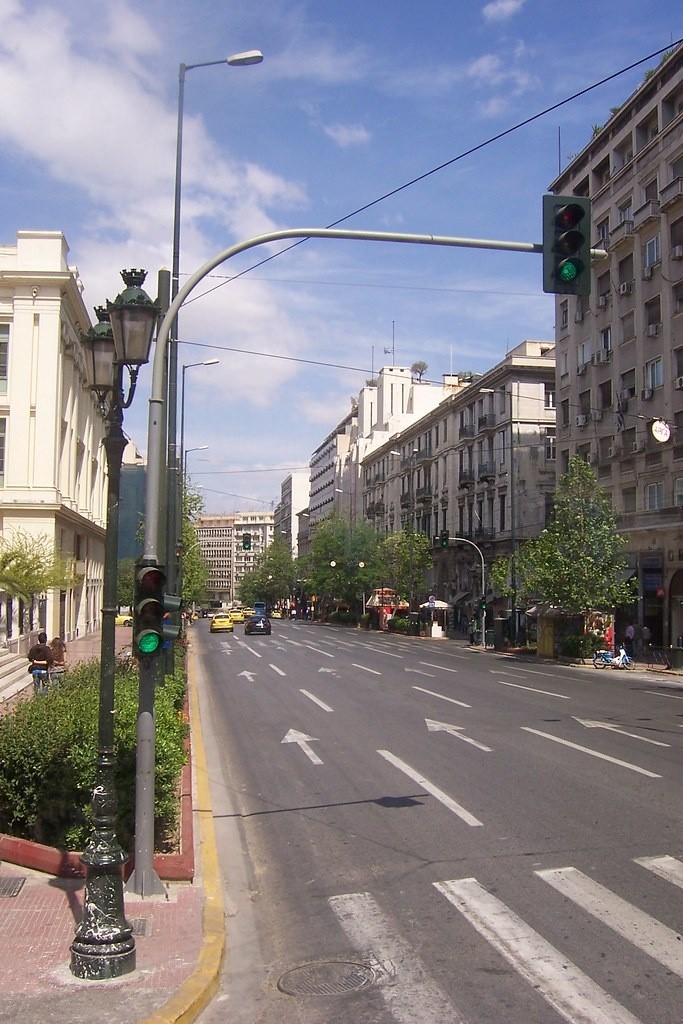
[461,615,480,646]
[625,621,650,657]
[282,607,296,620]
[29,633,67,695]
[182,608,193,620]
[306,607,310,621]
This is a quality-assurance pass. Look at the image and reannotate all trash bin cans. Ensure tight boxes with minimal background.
[672,649,683,668]
[485,630,494,646]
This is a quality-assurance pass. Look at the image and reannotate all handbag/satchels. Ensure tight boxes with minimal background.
[28,664,33,673]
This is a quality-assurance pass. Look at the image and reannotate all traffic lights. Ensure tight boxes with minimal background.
[162,592,183,642]
[440,530,450,549]
[481,594,486,610]
[131,562,168,664]
[541,193,593,297]
[242,533,251,550]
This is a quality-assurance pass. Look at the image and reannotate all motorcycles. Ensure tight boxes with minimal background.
[592,643,636,670]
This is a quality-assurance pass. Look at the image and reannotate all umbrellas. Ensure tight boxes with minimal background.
[420,600,452,610]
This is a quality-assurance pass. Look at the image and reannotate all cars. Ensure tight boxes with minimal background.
[114,614,133,627]
[208,614,234,633]
[272,609,283,619]
[243,614,272,635]
[201,600,268,624]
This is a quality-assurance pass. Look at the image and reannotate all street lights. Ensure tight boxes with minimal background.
[335,488,353,560]
[389,448,419,622]
[158,46,268,676]
[175,358,222,643]
[65,266,164,983]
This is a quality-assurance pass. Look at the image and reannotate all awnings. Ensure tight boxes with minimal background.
[467,592,503,608]
[448,592,470,605]
[589,569,636,602]
